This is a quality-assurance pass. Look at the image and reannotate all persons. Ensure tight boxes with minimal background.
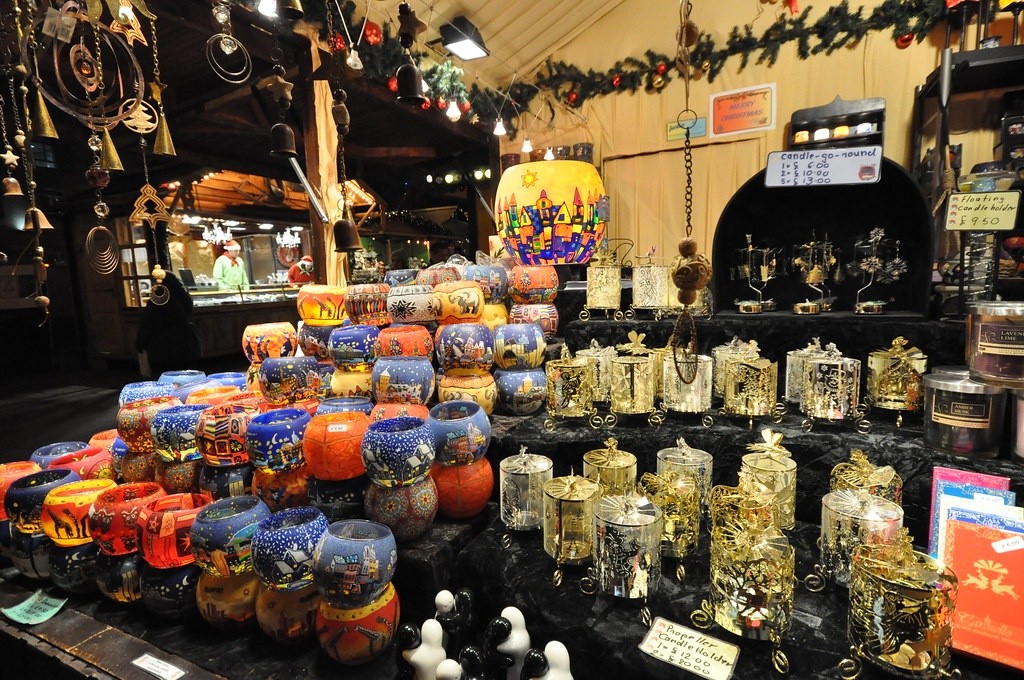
[288,255,315,288]
[135,271,200,382]
[213,239,250,291]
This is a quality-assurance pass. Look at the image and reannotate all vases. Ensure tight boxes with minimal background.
[0,145,627,667]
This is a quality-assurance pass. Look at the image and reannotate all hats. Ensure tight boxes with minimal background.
[301,255,313,266]
[223,240,241,250]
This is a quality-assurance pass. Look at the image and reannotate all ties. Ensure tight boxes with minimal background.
[223,251,239,266]
[298,261,314,276]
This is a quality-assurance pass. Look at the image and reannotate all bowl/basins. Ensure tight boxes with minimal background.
[255,279,269,285]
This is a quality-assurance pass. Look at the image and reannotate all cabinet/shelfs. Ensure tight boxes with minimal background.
[120,283,317,361]
[913,48,1024,316]
[0,309,1024,680]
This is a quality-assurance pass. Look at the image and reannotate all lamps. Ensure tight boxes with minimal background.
[424,15,492,60]
